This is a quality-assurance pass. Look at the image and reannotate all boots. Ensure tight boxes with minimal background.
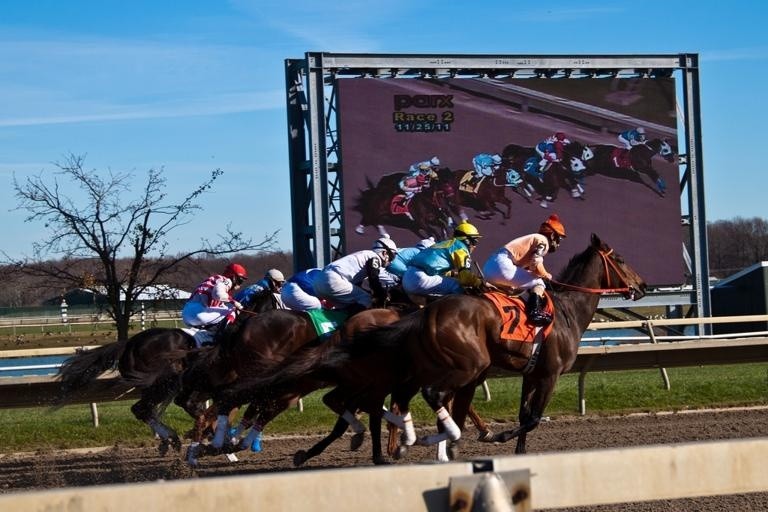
[528,292,553,324]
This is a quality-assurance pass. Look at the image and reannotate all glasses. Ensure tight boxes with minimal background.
[235,276,244,285]
[470,238,479,246]
[555,233,562,245]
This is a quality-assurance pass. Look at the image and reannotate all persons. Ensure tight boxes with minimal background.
[281,223,482,313]
[399,127,648,205]
[482,214,566,327]
[237,266,287,306]
[182,263,243,340]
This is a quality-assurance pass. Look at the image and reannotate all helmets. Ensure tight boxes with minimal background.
[539,213,565,237]
[222,262,249,281]
[453,220,482,238]
[416,236,435,247]
[373,234,398,260]
[265,267,286,285]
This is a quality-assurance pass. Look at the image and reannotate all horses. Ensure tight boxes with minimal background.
[40,232,650,467]
[346,137,676,244]
